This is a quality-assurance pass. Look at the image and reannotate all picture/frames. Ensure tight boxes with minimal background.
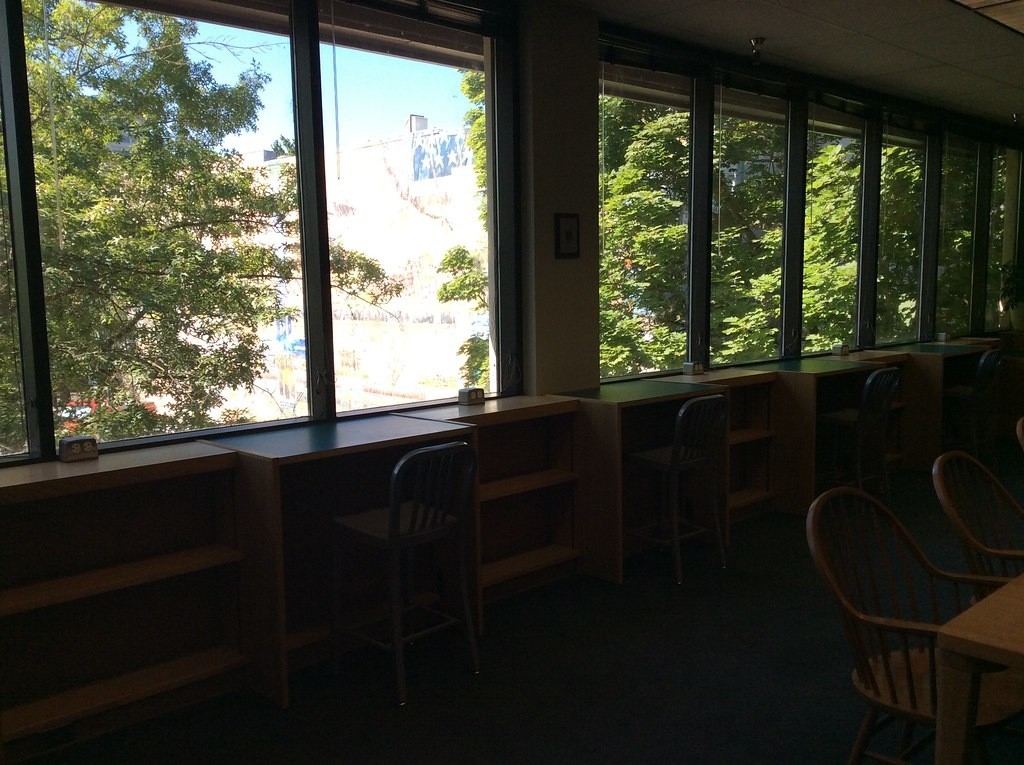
[554,211,581,261]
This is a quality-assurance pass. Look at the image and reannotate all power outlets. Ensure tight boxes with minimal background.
[59,436,99,463]
[937,332,951,341]
[833,344,851,357]
[459,387,487,405]
[684,361,706,376]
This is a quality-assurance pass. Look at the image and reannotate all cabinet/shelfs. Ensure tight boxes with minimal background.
[539,380,728,566]
[0,438,260,765]
[388,390,576,610]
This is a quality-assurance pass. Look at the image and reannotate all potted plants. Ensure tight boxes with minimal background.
[1002,257,1024,386]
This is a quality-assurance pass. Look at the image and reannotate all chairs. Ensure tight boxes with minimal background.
[799,485,1014,765]
[326,440,480,704]
[942,348,1004,460]
[817,365,906,519]
[618,392,728,583]
[933,449,1024,593]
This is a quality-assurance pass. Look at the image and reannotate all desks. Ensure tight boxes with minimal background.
[864,337,1001,474]
[823,349,909,474]
[936,569,1024,730]
[193,410,480,710]
[651,367,776,527]
